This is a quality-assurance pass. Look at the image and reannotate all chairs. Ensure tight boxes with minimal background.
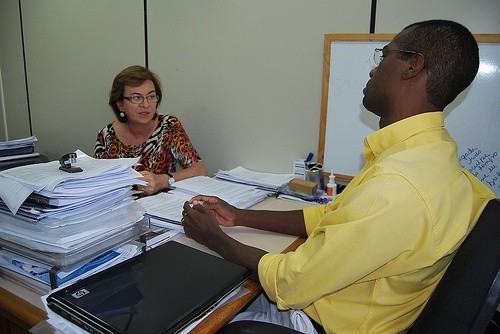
[219,198,500,334]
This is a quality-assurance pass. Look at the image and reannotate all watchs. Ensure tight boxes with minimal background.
[165,172,175,187]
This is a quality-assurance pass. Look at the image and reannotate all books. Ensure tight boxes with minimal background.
[0,135,301,334]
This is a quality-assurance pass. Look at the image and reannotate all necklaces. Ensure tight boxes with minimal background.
[114,121,155,145]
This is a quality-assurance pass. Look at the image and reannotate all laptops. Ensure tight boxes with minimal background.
[47,240,251,334]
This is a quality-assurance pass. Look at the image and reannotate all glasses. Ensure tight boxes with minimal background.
[373,48,414,67]
[124,95,159,105]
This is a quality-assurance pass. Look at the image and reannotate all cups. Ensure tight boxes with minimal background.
[304,162,325,190]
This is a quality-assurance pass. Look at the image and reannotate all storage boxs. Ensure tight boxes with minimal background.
[289,178,319,195]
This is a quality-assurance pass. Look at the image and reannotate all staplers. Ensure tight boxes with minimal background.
[59,152,84,173]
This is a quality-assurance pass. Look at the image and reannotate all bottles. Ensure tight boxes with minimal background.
[326,170,337,196]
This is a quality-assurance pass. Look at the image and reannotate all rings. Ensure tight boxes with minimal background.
[183,212,187,216]
[181,218,184,223]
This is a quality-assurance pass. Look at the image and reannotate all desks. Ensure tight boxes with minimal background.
[0,238,305,334]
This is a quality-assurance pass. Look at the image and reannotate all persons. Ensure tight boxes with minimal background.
[182,20,497,334]
[94,65,207,194]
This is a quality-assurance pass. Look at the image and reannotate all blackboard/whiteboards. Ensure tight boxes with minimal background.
[317,32,500,196]
